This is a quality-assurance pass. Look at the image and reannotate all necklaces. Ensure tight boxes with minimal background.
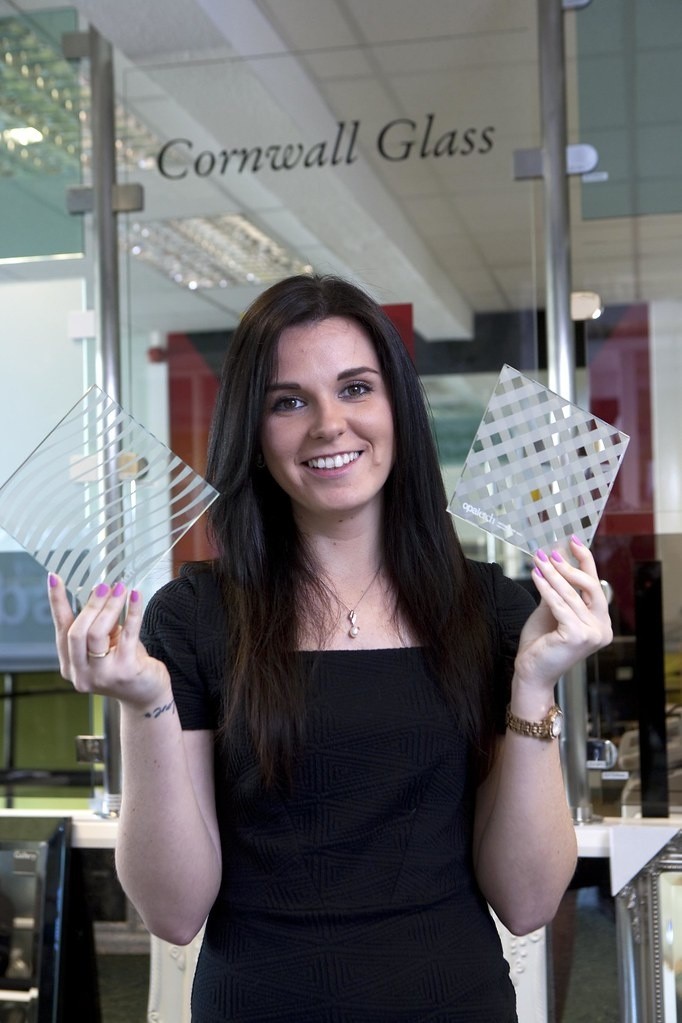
[320,566,381,638]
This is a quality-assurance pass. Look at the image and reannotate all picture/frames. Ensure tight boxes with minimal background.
[606,824,682,1023]
[0,814,74,1023]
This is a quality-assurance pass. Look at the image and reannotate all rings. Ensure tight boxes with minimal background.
[89,650,109,658]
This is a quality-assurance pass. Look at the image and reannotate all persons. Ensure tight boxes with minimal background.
[46,274,612,1023]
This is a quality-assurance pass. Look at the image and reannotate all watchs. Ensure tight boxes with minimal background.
[505,704,563,741]
